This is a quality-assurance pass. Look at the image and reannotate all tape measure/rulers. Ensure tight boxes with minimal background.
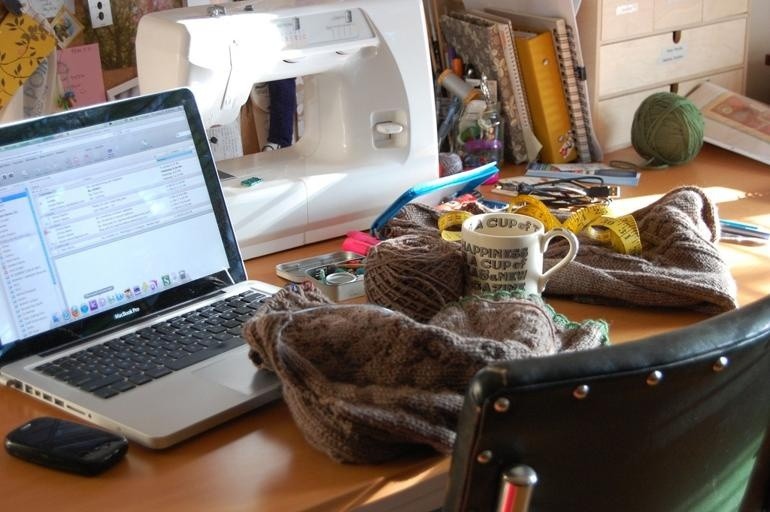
[438,196,644,255]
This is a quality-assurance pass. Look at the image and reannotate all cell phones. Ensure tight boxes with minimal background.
[2,414,130,474]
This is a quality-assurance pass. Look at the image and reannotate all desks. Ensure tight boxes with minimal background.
[0,144,770,512]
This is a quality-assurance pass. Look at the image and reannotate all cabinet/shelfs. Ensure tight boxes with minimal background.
[576,1,752,156]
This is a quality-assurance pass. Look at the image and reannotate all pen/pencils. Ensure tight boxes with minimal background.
[336,263,367,269]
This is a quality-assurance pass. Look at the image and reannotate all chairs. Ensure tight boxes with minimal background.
[442,297,770,512]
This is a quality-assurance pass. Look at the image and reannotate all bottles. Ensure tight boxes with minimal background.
[442,99,508,172]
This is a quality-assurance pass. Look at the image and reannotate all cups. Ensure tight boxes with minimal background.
[459,210,579,300]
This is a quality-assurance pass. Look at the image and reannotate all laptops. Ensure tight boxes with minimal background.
[0,86,281,453]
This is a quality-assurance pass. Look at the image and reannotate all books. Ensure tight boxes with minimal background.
[436,6,605,164]
